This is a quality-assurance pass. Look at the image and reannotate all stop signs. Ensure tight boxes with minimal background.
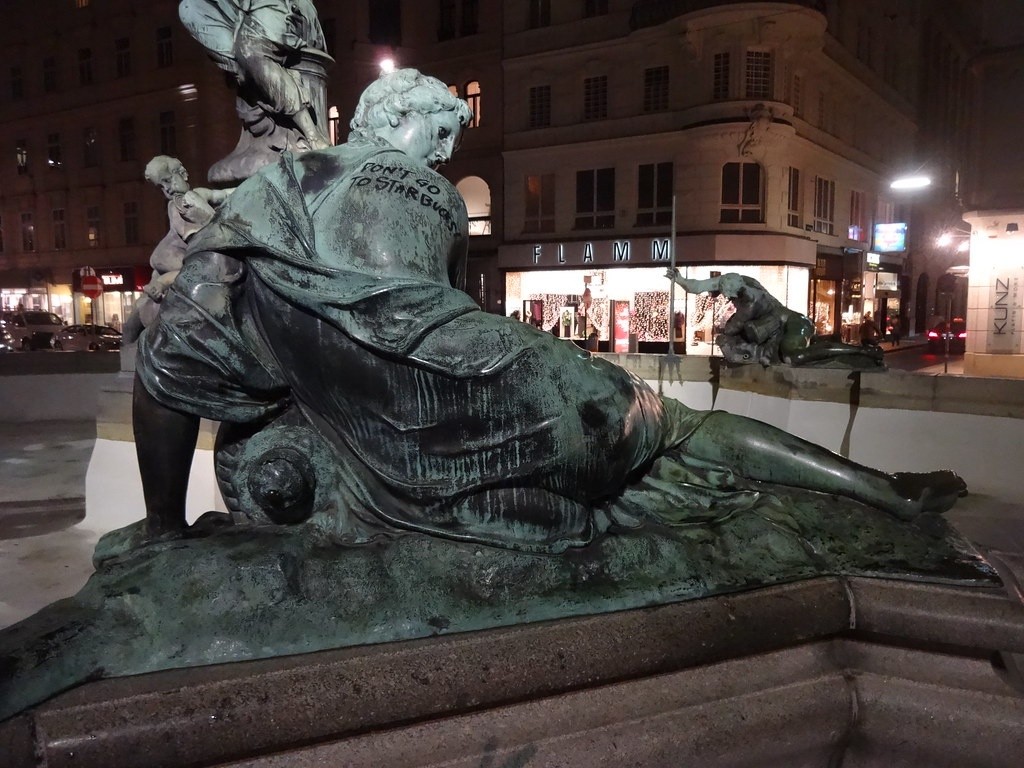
[82,276,103,299]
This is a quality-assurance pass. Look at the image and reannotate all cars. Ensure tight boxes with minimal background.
[929,321,966,353]
[49,324,122,351]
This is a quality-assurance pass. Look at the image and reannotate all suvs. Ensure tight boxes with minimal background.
[0,307,69,351]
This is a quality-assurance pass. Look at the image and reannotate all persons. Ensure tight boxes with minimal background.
[95,0,973,570]
[859,311,883,347]
[562,309,572,338]
[576,302,586,337]
[664,268,885,367]
[890,314,902,347]
[674,307,685,339]
